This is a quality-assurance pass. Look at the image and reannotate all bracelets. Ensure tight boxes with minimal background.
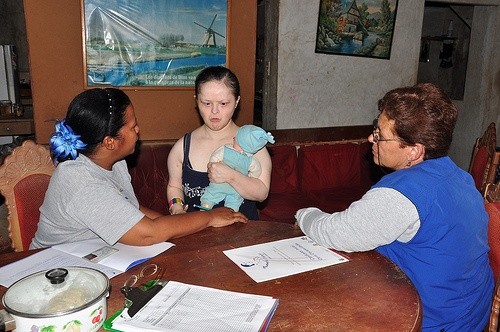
[169,198,185,207]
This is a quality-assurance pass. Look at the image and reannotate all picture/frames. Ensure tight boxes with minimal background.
[314,0,399,61]
[80,0,232,92]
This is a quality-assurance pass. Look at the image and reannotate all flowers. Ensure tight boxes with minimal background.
[50,119,87,161]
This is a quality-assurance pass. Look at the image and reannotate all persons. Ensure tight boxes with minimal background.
[199,124,275,213]
[293,85,495,332]
[28,88,249,251]
[166,66,273,221]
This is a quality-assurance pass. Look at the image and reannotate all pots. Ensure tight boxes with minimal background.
[0,266,110,332]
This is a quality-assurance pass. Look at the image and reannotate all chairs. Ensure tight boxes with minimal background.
[468,122,500,332]
[0,140,56,251]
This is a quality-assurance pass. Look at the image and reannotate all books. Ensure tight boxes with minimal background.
[0,238,177,289]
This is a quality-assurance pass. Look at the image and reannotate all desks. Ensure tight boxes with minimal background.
[0,224,423,332]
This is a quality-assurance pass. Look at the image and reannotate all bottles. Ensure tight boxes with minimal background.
[0,100,25,120]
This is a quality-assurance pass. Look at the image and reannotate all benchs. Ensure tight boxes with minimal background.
[129,123,397,225]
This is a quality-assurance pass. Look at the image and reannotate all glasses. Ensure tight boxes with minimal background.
[125,264,161,289]
[372,118,412,144]
